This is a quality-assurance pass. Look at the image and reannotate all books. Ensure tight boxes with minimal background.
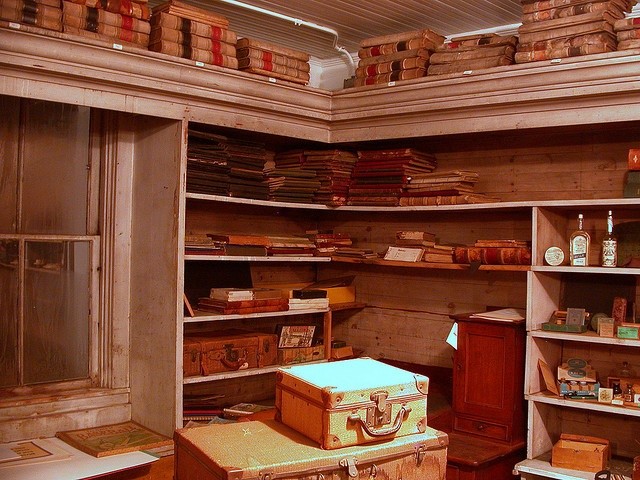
[277,147,358,207]
[427,32,518,76]
[0,439,74,469]
[613,16,640,51]
[347,149,437,206]
[514,0,637,62]
[288,279,356,311]
[400,167,500,205]
[384,228,531,263]
[198,288,289,315]
[156,0,239,70]
[235,38,311,86]
[264,167,321,205]
[307,227,378,259]
[185,232,269,257]
[185,395,276,427]
[62,0,153,52]
[268,233,316,258]
[354,28,447,89]
[1,1,62,33]
[188,128,271,200]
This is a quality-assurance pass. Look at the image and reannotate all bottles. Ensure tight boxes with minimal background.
[599,210,618,268]
[625,384,635,402]
[613,382,622,399]
[568,213,590,266]
[618,362,633,377]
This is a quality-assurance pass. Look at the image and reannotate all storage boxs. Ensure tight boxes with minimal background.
[552,434,612,472]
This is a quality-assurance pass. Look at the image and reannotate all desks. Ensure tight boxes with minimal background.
[427,388,527,479]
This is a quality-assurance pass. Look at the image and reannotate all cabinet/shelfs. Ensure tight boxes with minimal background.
[514,198,640,480]
[131,114,532,441]
[448,311,525,443]
